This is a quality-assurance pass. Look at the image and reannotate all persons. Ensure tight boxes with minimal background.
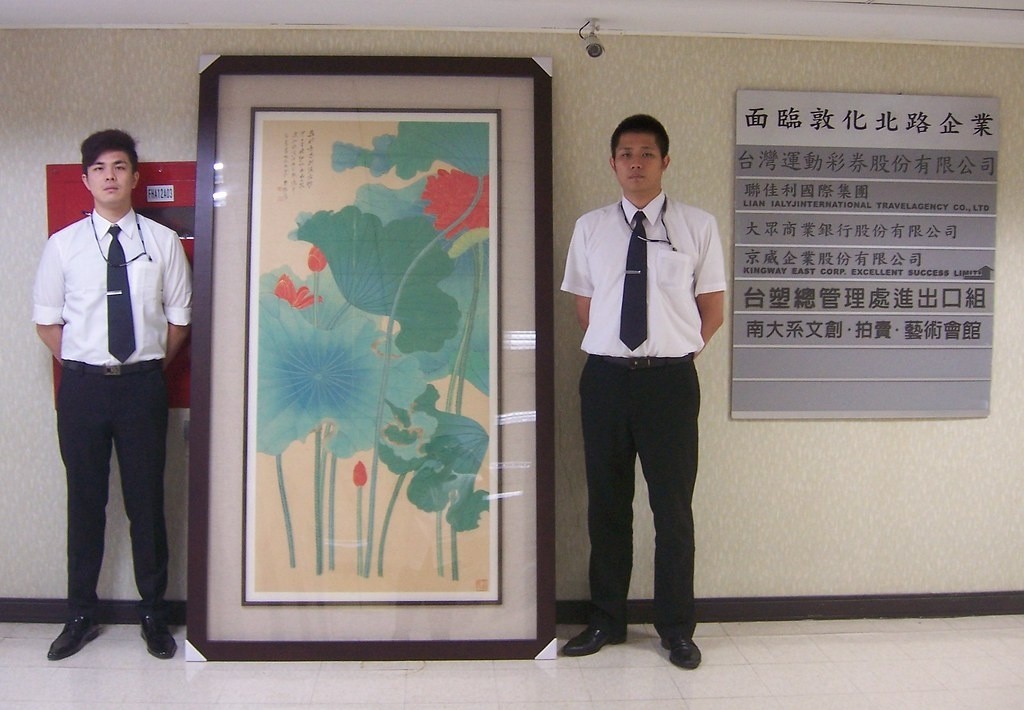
[33,128,191,660]
[561,113,725,669]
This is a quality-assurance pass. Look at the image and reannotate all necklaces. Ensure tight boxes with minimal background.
[90,204,152,266]
[619,200,677,251]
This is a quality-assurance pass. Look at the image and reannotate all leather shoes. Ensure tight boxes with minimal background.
[660,630,702,670]
[561,619,629,657]
[47,612,101,660]
[139,613,177,659]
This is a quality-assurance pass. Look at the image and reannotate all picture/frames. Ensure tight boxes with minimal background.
[184,55,557,662]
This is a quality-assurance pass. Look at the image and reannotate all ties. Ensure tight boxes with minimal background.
[107,225,136,363]
[618,210,649,353]
